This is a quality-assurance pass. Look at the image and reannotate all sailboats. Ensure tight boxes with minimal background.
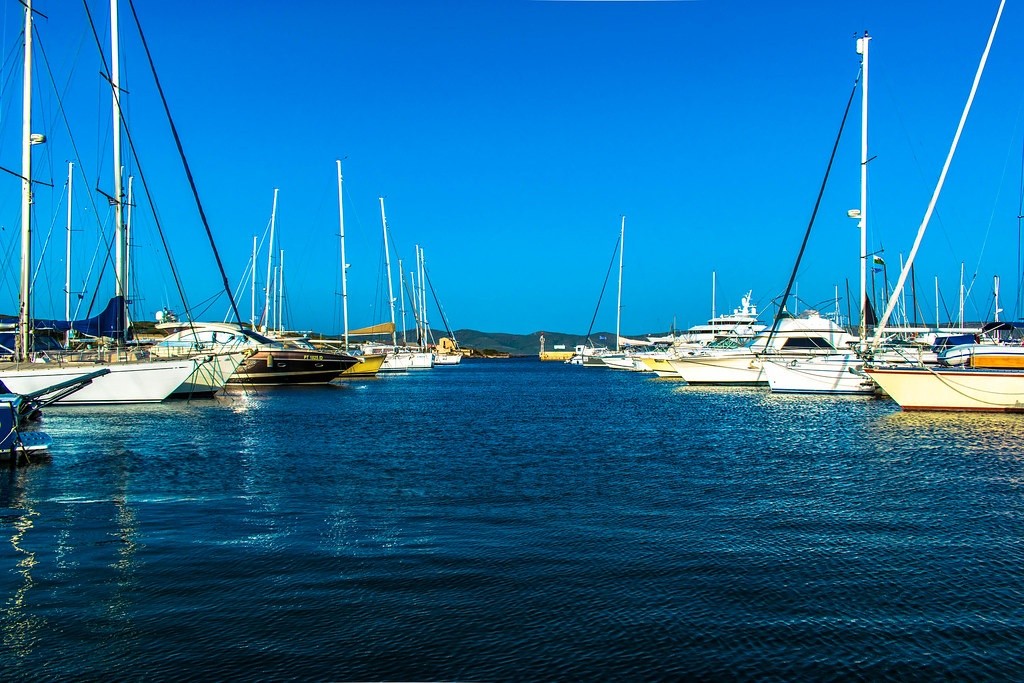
[540,26,1024,395]
[846,1,1024,411]
[0,0,463,458]
